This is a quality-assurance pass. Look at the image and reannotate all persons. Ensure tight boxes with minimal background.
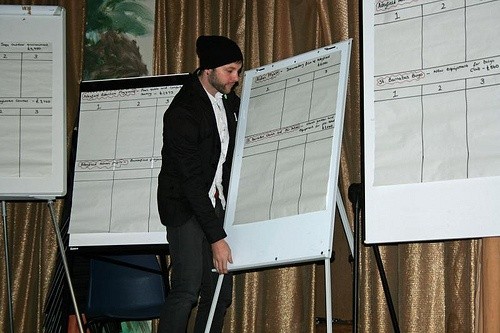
[156,34,243,333]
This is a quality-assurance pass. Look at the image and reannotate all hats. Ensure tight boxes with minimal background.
[196,35,243,70]
